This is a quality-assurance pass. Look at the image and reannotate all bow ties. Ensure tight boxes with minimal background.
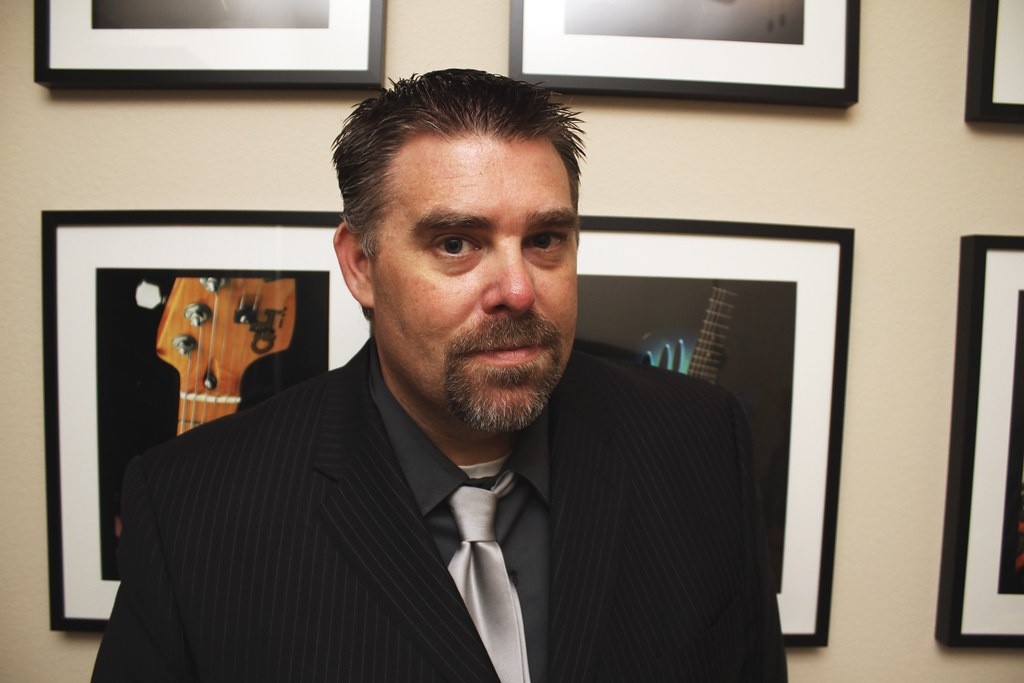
[447,470,534,681]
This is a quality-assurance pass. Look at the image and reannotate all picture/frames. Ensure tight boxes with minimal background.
[509,0,859,110]
[964,0,1024,123]
[34,0,387,96]
[39,210,856,648]
[935,233,1024,648]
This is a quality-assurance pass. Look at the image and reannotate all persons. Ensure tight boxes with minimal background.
[90,68,790,683]
